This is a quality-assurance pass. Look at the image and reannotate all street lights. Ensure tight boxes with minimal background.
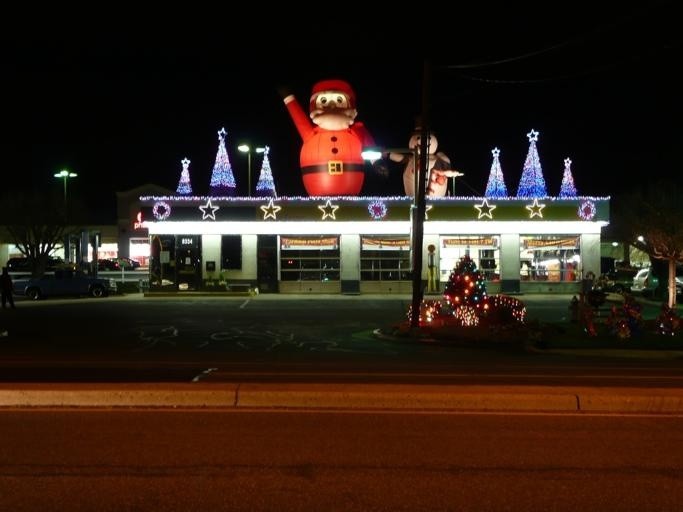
[237,145,266,196]
[52,167,81,261]
[360,146,419,272]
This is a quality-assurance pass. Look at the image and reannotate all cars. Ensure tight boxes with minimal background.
[600,256,682,305]
[5,254,140,301]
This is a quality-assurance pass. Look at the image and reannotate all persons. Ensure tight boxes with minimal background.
[0,267,16,309]
[282,79,389,197]
